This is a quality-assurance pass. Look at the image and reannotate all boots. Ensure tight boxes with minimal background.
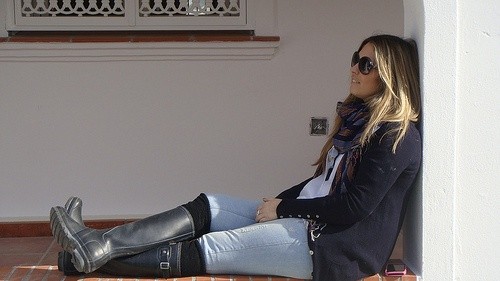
[49,205,194,274]
[58,196,182,277]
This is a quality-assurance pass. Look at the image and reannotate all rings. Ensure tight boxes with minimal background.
[258,210,260,214]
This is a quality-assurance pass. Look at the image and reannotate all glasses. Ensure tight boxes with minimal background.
[351,52,378,75]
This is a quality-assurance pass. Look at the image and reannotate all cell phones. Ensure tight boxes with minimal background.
[385,263,406,277]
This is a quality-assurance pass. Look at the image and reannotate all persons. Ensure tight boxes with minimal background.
[49,35,422,281]
